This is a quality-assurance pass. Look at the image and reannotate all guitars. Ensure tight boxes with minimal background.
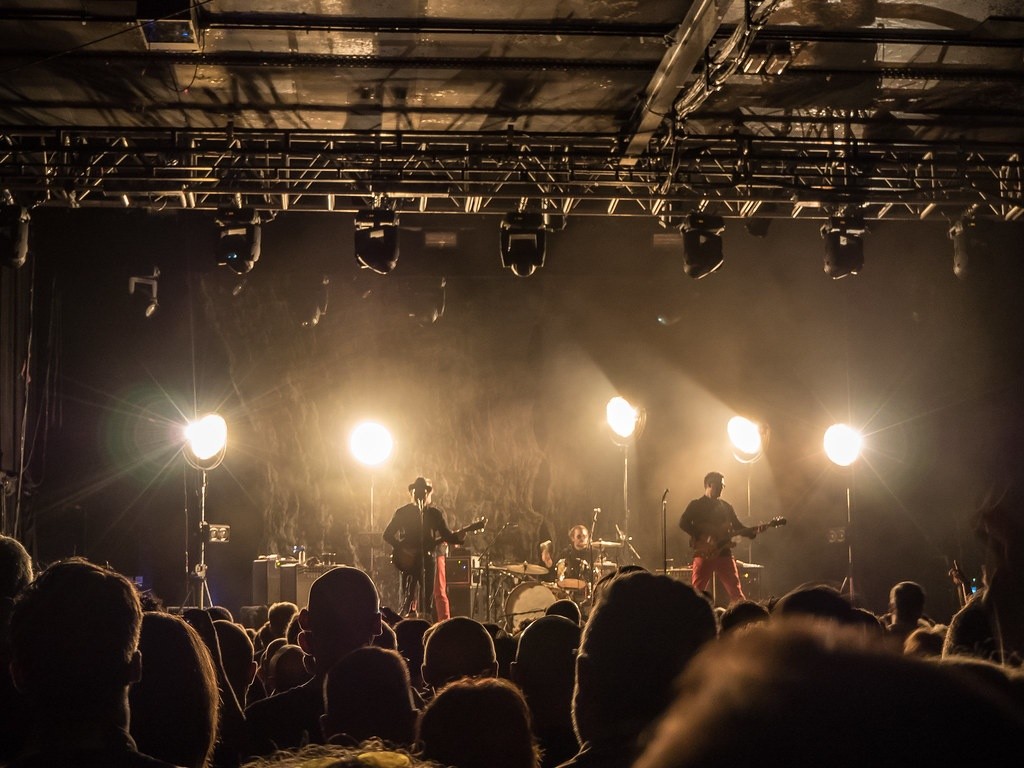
[391,517,488,572]
[691,516,788,560]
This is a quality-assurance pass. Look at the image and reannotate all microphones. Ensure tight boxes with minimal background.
[662,488,668,503]
[594,507,601,513]
[507,522,518,527]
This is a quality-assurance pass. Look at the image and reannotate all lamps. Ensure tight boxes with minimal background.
[0,200,31,272]
[217,207,262,271]
[811,203,871,283]
[680,211,727,279]
[353,204,400,274]
[945,210,1002,285]
[498,209,551,279]
[121,260,162,321]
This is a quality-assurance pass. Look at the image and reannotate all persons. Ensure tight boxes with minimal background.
[0,535,1024,768]
[679,472,768,606]
[384,478,467,620]
[540,524,599,581]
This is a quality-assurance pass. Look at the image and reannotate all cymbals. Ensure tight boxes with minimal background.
[474,566,507,571]
[505,560,549,575]
[591,541,620,547]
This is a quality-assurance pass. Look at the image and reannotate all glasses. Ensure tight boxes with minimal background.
[576,534,588,539]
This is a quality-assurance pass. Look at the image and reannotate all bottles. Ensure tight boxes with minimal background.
[298,547,305,563]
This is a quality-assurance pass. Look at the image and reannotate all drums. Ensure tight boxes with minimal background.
[555,557,591,590]
[592,563,617,583]
[543,582,572,600]
[504,582,557,636]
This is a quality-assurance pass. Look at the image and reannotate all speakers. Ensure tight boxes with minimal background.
[280,564,328,610]
[446,585,487,621]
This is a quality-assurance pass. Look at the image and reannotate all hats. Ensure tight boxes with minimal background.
[408,477,432,495]
